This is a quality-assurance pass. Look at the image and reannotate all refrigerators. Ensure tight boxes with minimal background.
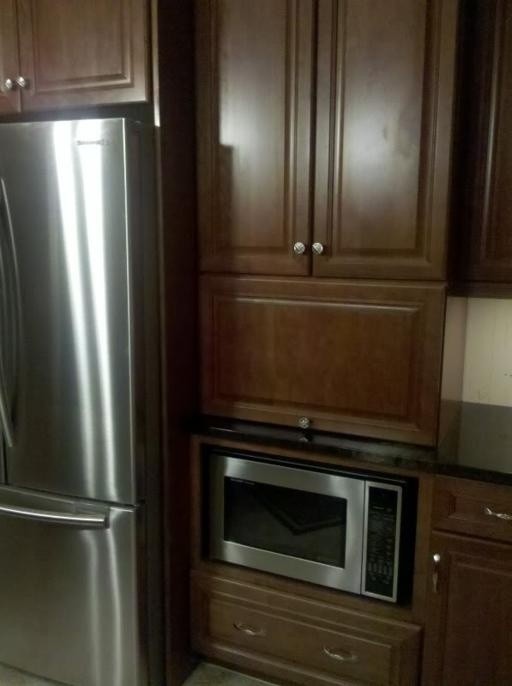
[0,114,166,686]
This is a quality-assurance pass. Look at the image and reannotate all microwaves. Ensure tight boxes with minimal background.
[204,449,414,605]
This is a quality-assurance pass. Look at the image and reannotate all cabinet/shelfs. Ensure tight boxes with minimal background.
[421,471,510,684]
[0,1,170,123]
[451,1,511,297]
